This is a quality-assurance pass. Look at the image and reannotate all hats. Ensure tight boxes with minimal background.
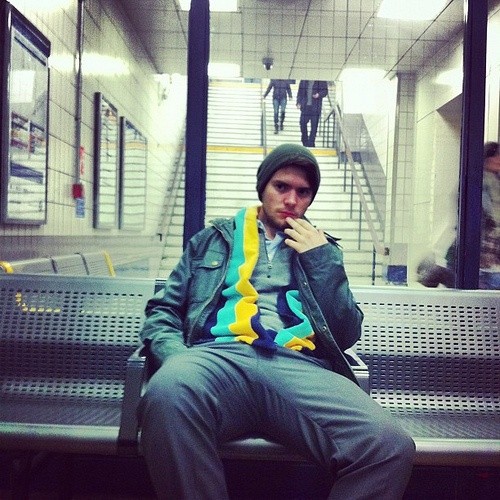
[256,144,321,206]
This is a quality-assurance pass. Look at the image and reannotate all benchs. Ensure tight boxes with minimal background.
[0,250,116,312]
[0,272,500,500]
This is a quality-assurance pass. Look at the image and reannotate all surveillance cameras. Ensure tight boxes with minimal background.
[262,57,274,70]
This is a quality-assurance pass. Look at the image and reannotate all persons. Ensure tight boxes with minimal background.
[296,80,328,147]
[139,144,416,500]
[264,80,293,134]
[479,142,500,290]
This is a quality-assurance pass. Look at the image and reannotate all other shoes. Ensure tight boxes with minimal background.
[275,125,278,134]
[280,124,283,130]
